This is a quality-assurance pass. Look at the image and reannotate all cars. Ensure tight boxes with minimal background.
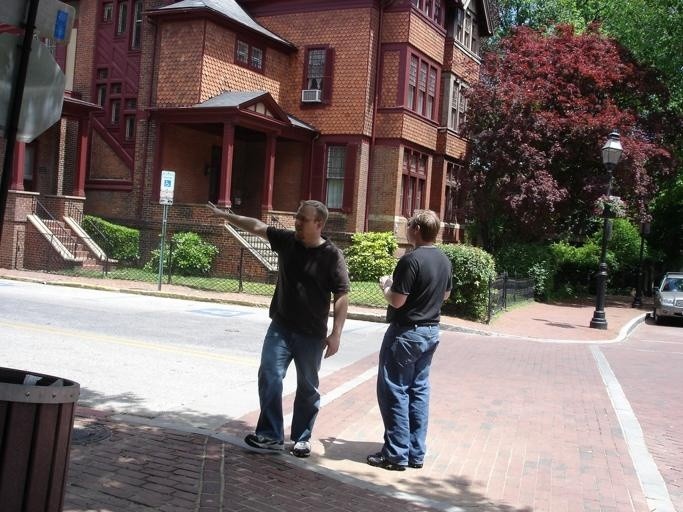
[654,272,683,322]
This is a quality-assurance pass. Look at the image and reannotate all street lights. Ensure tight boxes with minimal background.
[590,128,623,329]
[631,221,650,309]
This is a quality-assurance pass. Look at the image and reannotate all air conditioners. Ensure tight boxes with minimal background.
[301,88,320,104]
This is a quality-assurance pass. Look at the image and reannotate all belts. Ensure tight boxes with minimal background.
[404,319,439,326]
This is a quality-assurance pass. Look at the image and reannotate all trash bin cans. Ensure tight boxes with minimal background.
[0,366,80,512]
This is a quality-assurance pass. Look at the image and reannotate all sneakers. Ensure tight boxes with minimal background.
[366,448,426,472]
[243,434,285,450]
[292,440,311,456]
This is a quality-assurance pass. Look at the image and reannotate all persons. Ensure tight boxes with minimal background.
[365,207,454,472]
[202,198,352,458]
